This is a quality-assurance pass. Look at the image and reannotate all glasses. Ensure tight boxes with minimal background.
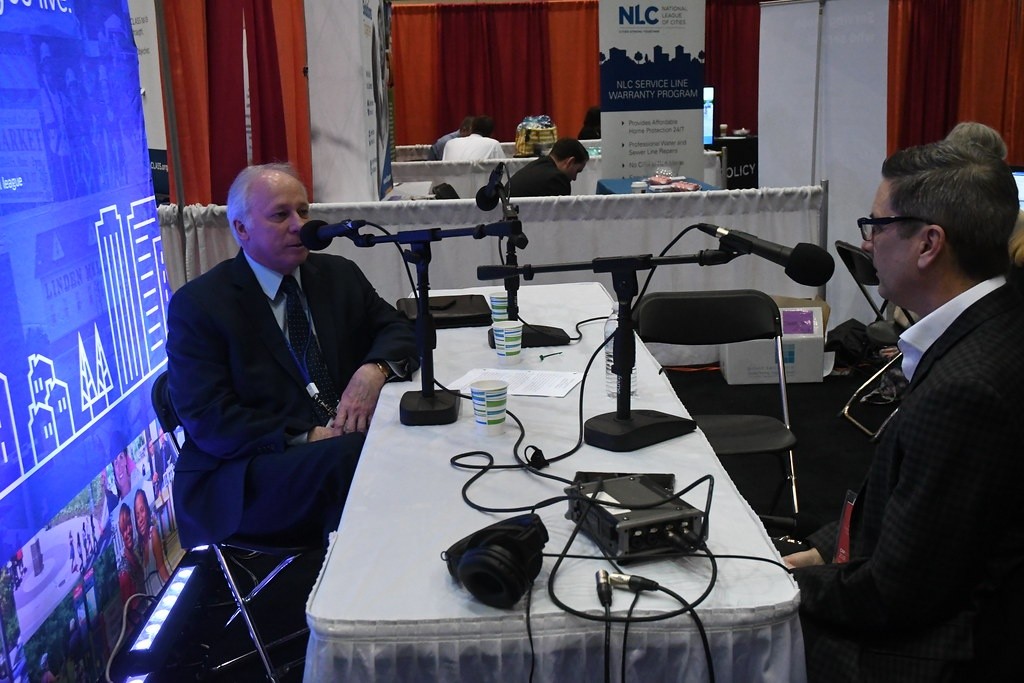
[857,217,949,241]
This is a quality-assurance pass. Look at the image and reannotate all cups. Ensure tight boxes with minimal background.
[631,181,648,195]
[489,291,509,322]
[719,124,728,136]
[469,379,509,436]
[491,321,524,367]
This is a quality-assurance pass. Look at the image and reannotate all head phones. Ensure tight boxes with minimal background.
[445,513,549,609]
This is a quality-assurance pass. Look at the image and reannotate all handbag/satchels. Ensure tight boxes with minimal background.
[859,369,908,406]
[515,123,558,156]
[396,295,492,329]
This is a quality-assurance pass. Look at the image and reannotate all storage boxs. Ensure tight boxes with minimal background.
[596,178,726,191]
[719,294,830,386]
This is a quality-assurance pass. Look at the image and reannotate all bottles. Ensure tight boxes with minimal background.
[604,301,638,398]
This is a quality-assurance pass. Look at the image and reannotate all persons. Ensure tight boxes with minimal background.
[7,548,29,591]
[35,43,137,200]
[99,466,121,515]
[578,103,602,140]
[148,426,175,501]
[134,490,169,606]
[110,447,151,502]
[68,514,98,573]
[428,114,507,160]
[166,160,421,548]
[778,121,1024,683]
[118,502,149,630]
[505,136,589,197]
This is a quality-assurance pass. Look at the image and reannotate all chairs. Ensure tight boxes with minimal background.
[147,373,308,683]
[834,240,918,437]
[637,289,800,531]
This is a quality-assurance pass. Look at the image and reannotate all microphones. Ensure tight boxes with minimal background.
[697,222,835,287]
[476,163,504,211]
[300,219,366,251]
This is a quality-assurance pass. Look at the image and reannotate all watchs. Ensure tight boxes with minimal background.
[375,361,390,384]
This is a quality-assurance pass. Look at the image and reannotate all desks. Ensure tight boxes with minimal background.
[303,282,806,683]
[711,136,758,189]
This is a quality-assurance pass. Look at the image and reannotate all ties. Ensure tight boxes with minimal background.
[278,273,341,427]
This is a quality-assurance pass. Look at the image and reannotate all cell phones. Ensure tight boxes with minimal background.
[572,471,675,494]
